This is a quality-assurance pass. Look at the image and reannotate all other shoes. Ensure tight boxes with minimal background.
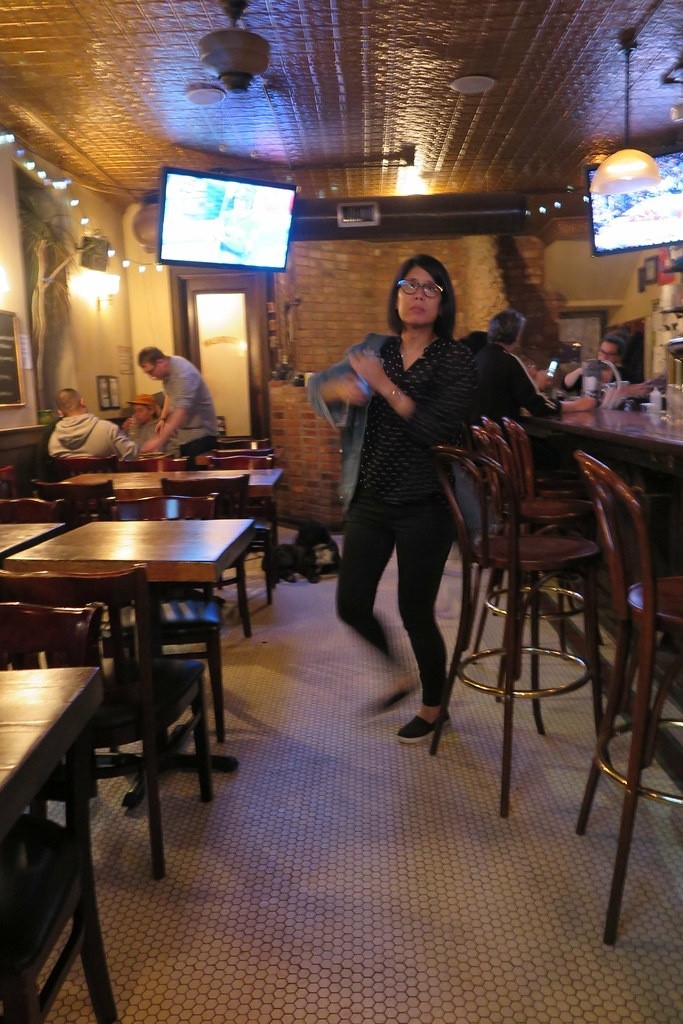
[371,680,415,717]
[396,708,452,744]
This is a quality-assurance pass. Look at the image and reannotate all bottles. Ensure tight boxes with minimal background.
[648,385,663,411]
[128,415,137,436]
[279,354,293,379]
[305,371,315,387]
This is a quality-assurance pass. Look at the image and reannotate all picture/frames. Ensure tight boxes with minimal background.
[643,255,659,285]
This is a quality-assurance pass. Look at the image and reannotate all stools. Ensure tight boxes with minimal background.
[429,415,683,945]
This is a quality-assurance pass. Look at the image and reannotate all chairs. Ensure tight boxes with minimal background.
[0,436,277,1024]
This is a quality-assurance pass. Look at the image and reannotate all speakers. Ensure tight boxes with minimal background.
[81,237,109,272]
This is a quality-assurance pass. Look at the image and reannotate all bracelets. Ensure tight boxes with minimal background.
[158,417,166,421]
[386,385,407,405]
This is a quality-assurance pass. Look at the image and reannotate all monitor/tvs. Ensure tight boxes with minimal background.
[156,166,298,272]
[586,147,683,257]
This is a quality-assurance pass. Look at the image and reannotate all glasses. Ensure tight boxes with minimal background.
[597,347,619,360]
[397,279,445,298]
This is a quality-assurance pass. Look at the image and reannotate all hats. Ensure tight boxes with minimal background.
[124,393,156,407]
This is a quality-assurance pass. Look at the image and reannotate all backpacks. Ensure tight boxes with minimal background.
[296,516,342,575]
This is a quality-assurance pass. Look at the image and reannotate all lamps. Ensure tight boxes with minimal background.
[589,38,661,193]
[132,191,159,253]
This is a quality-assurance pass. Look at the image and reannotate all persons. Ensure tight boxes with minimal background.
[306,252,479,744]
[562,332,647,411]
[46,385,140,462]
[465,307,595,455]
[137,347,218,459]
[119,392,165,456]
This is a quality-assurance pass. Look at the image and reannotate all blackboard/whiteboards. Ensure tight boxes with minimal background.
[0,309,26,409]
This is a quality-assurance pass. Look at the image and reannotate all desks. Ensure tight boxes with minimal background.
[5,519,256,807]
[0,667,116,1024]
[59,468,283,604]
[0,522,64,559]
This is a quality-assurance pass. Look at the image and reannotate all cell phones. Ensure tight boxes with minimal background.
[546,361,558,378]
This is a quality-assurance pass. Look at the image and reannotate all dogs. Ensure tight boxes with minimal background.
[262,524,341,584]
